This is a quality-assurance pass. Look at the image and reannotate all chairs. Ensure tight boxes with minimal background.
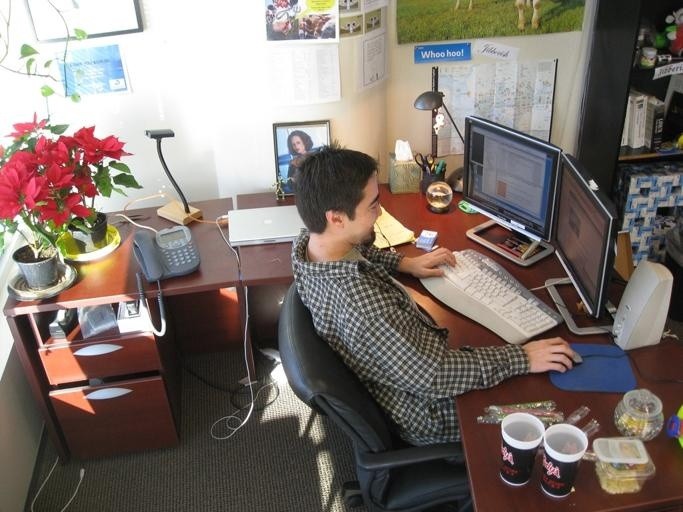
[276,278,474,511]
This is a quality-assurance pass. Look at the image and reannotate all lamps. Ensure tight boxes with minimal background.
[412,89,464,193]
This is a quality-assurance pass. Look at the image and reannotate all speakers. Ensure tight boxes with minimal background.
[612,260,673,350]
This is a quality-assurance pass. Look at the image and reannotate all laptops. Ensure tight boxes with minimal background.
[228,205,311,247]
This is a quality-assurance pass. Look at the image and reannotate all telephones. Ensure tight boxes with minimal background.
[132,226,200,283]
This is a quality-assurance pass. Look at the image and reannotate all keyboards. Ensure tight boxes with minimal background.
[419,248,564,345]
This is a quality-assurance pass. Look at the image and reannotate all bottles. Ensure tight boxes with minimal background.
[633,27,657,71]
[612,387,666,442]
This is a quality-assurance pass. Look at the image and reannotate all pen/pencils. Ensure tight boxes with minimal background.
[422,154,446,175]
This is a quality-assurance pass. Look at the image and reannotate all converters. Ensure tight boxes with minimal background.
[262,348,282,363]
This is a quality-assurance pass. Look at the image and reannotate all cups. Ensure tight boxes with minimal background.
[539,424,587,500]
[422,170,445,196]
[501,412,544,488]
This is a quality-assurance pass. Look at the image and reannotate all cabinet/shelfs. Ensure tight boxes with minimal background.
[577,1,683,287]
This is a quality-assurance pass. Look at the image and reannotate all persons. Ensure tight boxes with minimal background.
[287,130,313,189]
[290,141,583,468]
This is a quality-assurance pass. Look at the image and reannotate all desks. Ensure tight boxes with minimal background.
[0,176,682,511]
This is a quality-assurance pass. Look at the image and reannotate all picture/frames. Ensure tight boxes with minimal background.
[22,1,143,46]
[271,119,331,200]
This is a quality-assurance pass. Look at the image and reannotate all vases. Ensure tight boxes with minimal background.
[12,242,59,291]
[65,211,108,255]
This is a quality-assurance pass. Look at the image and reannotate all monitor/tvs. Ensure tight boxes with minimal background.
[462,116,562,266]
[546,153,617,335]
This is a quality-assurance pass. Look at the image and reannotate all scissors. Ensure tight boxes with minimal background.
[415,153,434,175]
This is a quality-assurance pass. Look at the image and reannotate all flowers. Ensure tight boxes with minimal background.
[0,135,90,263]
[4,108,142,236]
